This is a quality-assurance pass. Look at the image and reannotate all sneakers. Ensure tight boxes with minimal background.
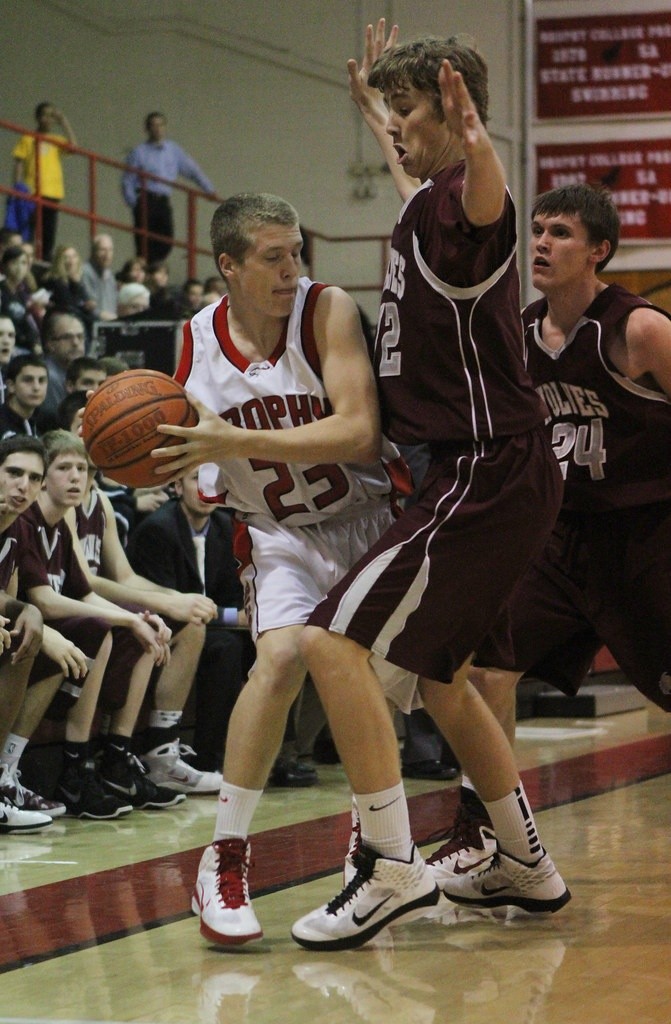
[191,955,264,1022]
[0,800,54,834]
[52,760,133,818]
[96,743,186,809]
[443,838,572,915]
[427,802,506,877]
[446,922,564,981]
[291,841,441,950]
[139,737,224,792]
[344,797,363,887]
[292,952,443,1022]
[191,836,264,949]
[0,759,67,817]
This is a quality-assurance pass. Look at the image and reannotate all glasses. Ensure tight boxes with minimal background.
[51,332,87,344]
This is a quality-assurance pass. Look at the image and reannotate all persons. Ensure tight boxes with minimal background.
[10,102,78,260]
[121,111,218,264]
[428,184,671,891]
[76,191,403,946]
[0,230,457,834]
[289,17,572,952]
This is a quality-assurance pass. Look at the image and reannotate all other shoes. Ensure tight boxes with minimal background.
[403,759,458,781]
[268,760,319,787]
[311,737,340,764]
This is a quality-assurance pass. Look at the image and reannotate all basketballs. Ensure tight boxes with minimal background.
[82,369,197,488]
